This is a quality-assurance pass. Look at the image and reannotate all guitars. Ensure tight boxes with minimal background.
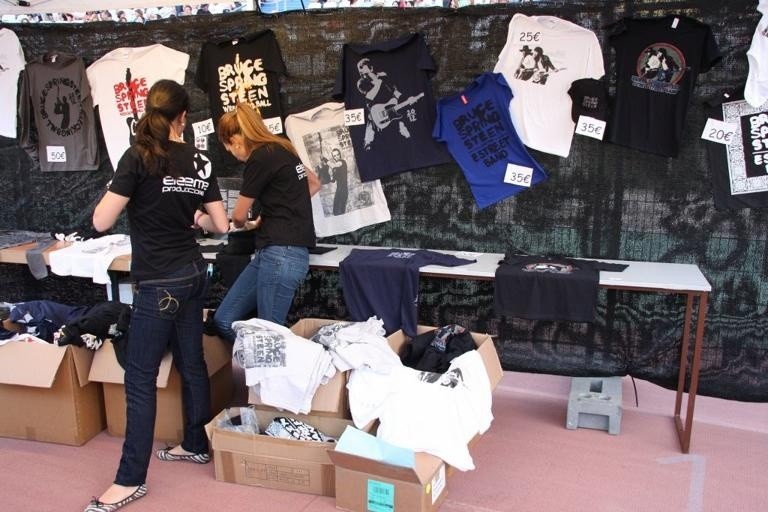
[658,67,691,81]
[315,132,330,185]
[371,93,425,129]
[532,68,566,83]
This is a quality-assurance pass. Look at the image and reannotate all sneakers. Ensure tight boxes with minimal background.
[84,483,147,512]
[155,447,210,465]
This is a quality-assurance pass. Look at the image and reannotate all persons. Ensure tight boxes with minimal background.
[321,148,348,216]
[213,103,322,344]
[641,47,682,83]
[514,44,559,85]
[84,80,229,512]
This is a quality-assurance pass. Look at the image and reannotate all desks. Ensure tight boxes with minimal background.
[305,239,713,451]
[3,232,148,309]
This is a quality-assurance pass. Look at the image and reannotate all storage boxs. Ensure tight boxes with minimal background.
[247,316,359,421]
[373,323,503,434]
[205,407,450,512]
[2,301,104,446]
[89,328,234,445]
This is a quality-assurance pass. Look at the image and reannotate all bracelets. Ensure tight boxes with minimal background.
[196,214,204,225]
